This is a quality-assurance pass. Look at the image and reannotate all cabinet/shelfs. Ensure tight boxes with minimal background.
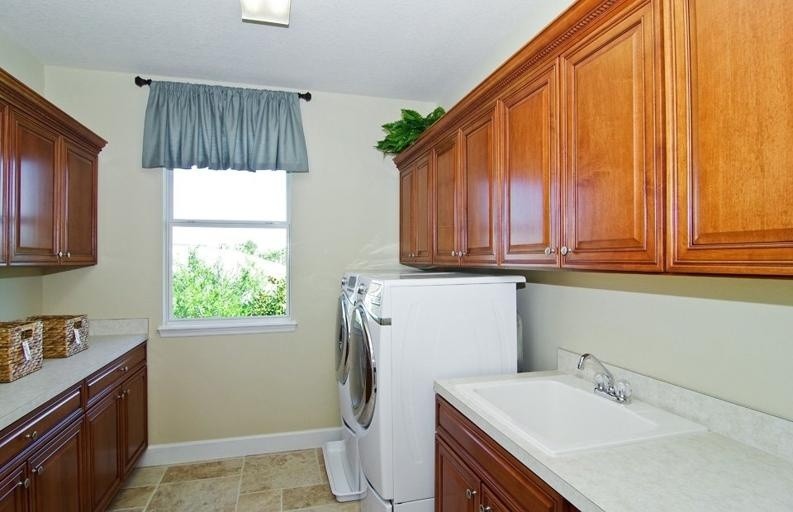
[0,381,84,512]
[500,1,662,272]
[663,0,793,277]
[84,341,148,512]
[393,126,431,265]
[432,68,500,266]
[435,394,580,512]
[0,67,108,267]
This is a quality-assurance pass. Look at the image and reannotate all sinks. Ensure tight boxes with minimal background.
[473,380,663,453]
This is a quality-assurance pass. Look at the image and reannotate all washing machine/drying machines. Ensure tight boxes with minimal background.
[321,272,527,512]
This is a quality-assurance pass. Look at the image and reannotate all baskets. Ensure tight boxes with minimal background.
[1,320,43,384]
[27,314,91,359]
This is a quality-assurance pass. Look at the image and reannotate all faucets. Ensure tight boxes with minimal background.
[577,353,614,393]
